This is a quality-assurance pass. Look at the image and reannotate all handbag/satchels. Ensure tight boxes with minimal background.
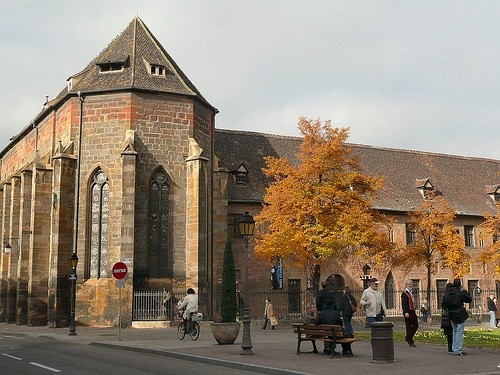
[345,294,358,312]
[441,318,451,328]
[270,316,279,326]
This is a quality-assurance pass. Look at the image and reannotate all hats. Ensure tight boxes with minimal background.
[370,278,380,283]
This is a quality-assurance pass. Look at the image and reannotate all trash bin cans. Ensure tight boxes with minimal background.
[370,322,395,361]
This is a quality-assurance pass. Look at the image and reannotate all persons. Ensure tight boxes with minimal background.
[162,290,179,320]
[440,279,472,356]
[178,288,198,336]
[401,279,419,347]
[360,278,387,348]
[420,297,432,327]
[483,294,500,328]
[261,298,275,330]
[309,281,358,355]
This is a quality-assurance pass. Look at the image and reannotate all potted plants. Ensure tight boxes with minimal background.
[211,236,241,345]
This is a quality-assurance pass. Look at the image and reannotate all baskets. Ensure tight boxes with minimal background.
[178,309,184,317]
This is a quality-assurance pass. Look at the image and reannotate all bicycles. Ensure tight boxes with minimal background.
[177,309,200,341]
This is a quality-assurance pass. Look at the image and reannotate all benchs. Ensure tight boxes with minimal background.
[290,322,361,359]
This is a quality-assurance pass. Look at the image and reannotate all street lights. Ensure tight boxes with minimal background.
[68,252,79,336]
[238,210,257,355]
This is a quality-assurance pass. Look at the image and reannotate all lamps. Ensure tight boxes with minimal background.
[4,237,20,255]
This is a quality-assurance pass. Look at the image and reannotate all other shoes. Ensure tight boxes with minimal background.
[323,349,329,353]
[448,350,453,354]
[193,332,197,335]
[183,328,187,330]
[493,326,498,329]
[405,336,407,341]
[343,352,349,355]
[272,328,275,329]
[454,352,467,356]
[410,344,416,347]
[327,350,340,355]
[351,333,354,338]
[262,327,265,329]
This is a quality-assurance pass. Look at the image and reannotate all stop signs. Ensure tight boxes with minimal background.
[112,261,128,280]
